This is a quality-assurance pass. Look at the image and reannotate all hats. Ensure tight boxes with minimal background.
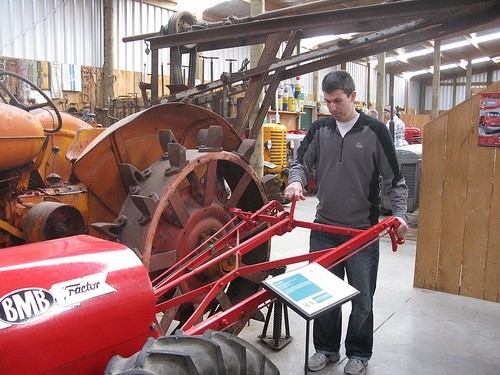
[384,106,397,113]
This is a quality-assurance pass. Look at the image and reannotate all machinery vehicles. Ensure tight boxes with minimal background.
[0,70,404,375]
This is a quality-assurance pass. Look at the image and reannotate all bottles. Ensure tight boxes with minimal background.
[278,76,301,112]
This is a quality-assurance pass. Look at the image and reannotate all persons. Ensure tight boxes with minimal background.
[383,106,405,146]
[284,69,410,375]
[368,109,379,120]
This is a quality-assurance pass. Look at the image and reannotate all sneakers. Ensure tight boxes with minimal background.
[308,352,341,371]
[344,359,368,375]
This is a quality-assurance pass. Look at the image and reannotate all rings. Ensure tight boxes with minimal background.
[286,193,289,196]
